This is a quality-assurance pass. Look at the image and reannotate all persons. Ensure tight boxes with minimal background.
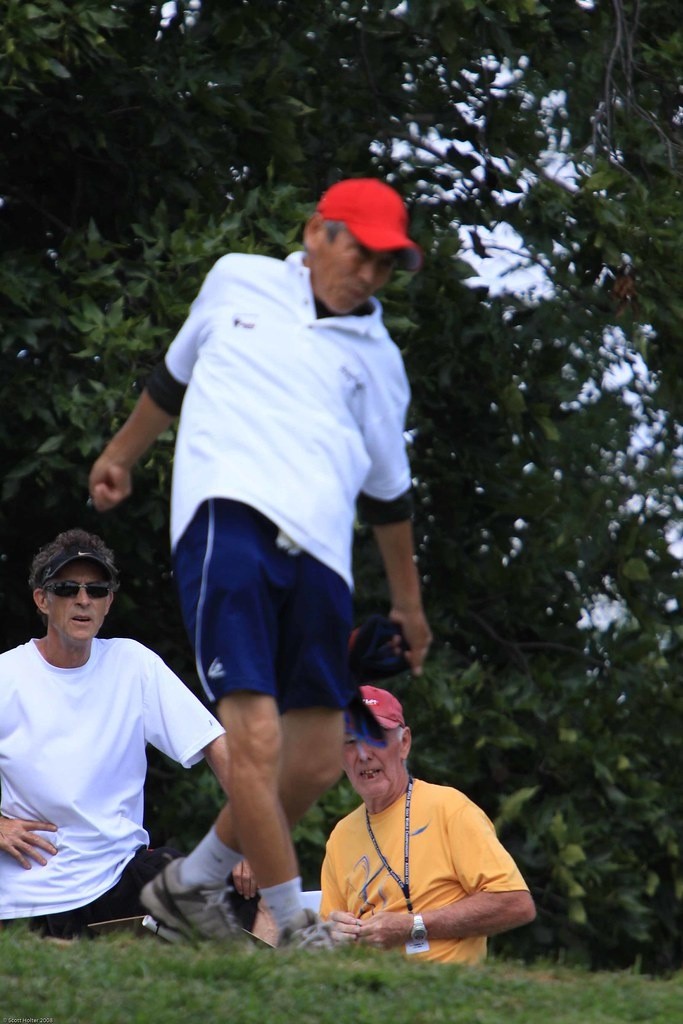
[90,175,434,956]
[311,688,536,968]
[0,528,278,955]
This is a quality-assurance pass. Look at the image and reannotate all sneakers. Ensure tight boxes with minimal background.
[140,856,256,957]
[274,908,334,962]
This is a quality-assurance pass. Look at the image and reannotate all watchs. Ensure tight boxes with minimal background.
[409,913,428,944]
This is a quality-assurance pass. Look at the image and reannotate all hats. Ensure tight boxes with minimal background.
[342,682,404,731]
[39,545,117,588]
[320,177,423,272]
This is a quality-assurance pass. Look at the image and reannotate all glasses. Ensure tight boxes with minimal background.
[37,579,110,598]
[342,618,388,749]
